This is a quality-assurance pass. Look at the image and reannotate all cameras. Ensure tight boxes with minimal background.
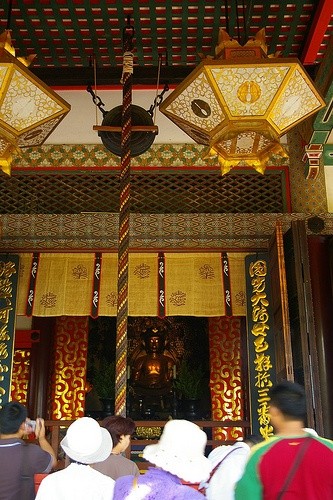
[24,419,37,432]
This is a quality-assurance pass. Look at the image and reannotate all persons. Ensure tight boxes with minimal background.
[114,419,210,500]
[205,439,255,500]
[235,381,333,500]
[0,402,55,500]
[134,322,176,389]
[89,415,141,481]
[34,417,115,500]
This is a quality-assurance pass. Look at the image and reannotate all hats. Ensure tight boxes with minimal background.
[60,416,113,465]
[142,420,211,484]
